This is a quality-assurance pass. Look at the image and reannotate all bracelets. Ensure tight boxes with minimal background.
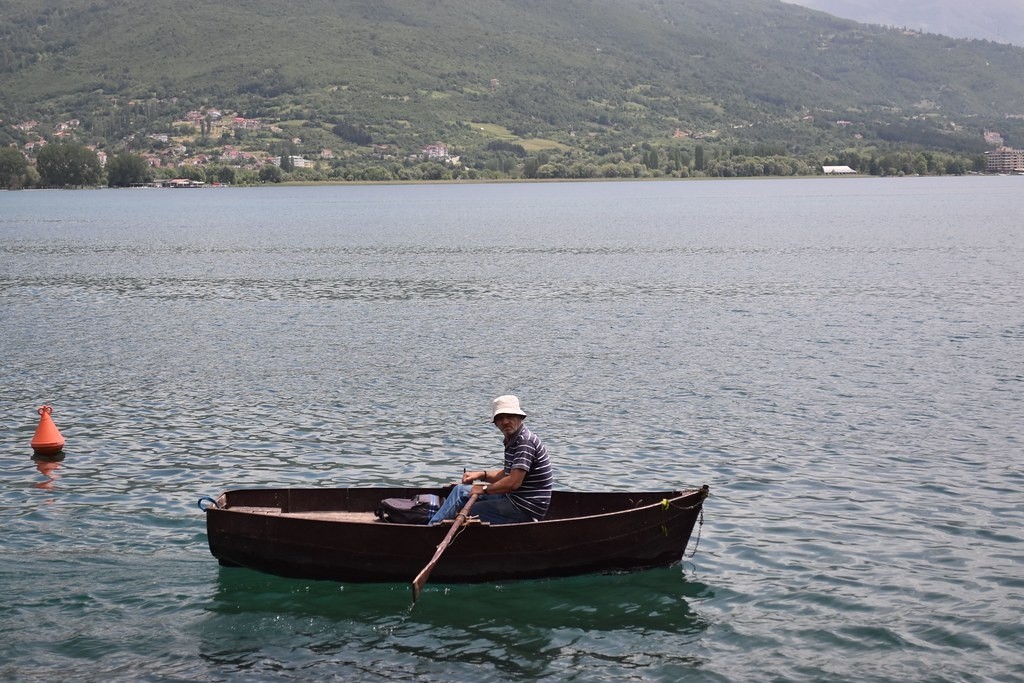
[482,470,487,482]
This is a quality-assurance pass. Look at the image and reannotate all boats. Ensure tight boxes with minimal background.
[200,483,712,590]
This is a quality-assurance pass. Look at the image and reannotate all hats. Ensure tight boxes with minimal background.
[490,395,527,423]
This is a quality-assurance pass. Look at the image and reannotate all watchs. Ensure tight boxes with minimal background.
[483,485,489,494]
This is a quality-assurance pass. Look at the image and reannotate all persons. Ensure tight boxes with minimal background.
[427,395,553,525]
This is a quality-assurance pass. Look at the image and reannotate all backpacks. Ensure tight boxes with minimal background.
[379,494,446,523]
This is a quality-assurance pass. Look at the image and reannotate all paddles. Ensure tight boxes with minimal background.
[411,492,479,604]
